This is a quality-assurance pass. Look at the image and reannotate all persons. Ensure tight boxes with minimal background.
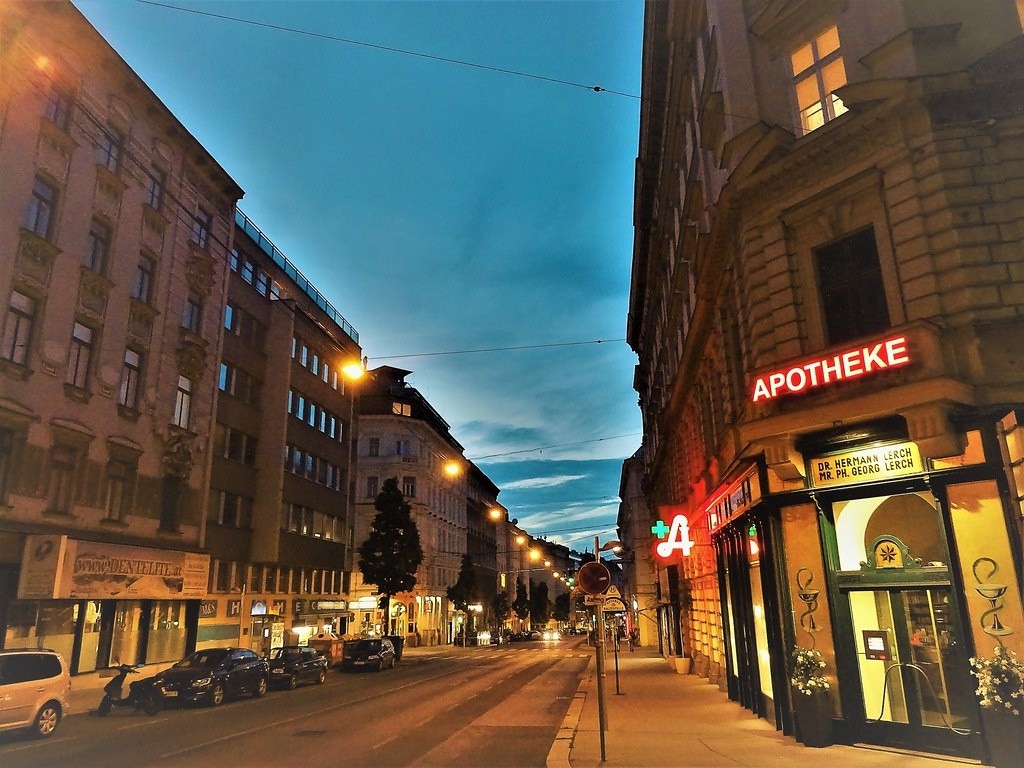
[617,629,638,646]
[628,629,635,652]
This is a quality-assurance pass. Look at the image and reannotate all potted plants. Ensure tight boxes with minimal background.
[674,578,693,674]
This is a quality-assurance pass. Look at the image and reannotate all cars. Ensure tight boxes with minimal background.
[343,638,396,672]
[154,647,269,707]
[261,645,329,692]
[506,626,587,642]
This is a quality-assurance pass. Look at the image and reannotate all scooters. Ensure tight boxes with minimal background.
[96,655,167,716]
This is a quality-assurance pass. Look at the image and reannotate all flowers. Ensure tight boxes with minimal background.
[968,648,1024,719]
[791,644,832,695]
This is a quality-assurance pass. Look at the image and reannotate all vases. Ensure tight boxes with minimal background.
[981,710,1024,768]
[792,688,836,748]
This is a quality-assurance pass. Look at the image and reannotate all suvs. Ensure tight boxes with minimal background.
[0,647,73,738]
